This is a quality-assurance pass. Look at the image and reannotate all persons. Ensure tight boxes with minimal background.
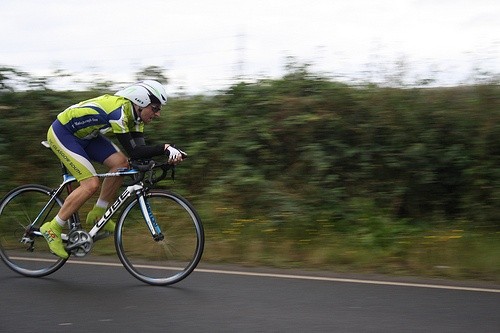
[39,81,187,260]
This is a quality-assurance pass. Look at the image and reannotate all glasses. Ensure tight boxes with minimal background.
[150,104,161,113]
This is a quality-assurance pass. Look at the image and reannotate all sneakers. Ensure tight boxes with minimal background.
[86,204,124,234]
[40,219,69,259]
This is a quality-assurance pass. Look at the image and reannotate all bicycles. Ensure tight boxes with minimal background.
[1,140,204,287]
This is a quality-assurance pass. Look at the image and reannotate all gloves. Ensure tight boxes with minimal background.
[164,143,188,165]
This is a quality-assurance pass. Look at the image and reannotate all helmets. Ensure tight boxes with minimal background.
[113,80,168,108]
